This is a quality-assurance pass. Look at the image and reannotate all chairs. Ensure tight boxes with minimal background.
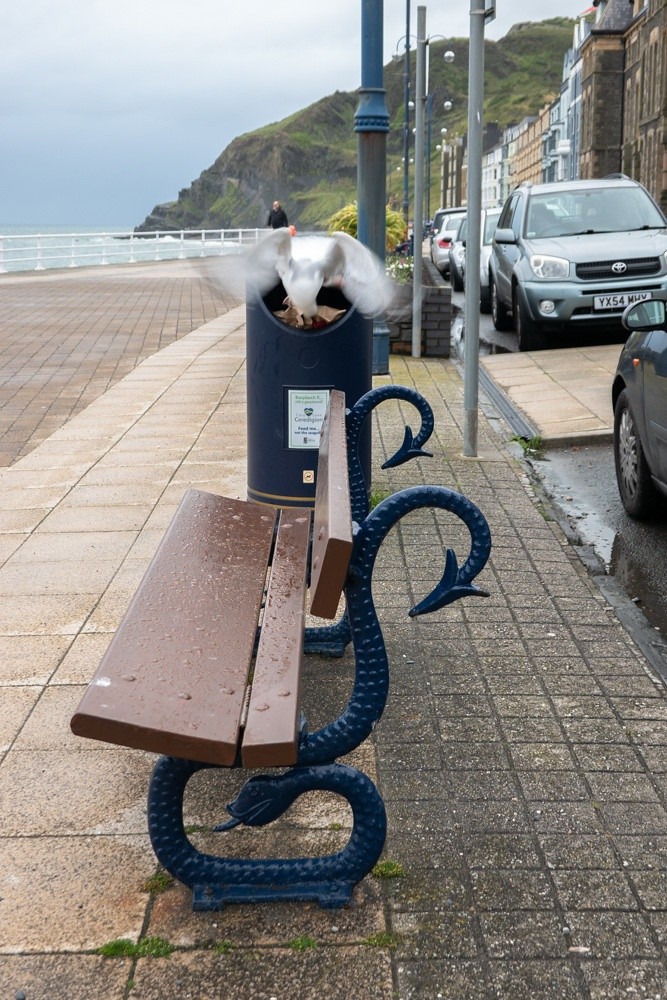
[528,209,556,233]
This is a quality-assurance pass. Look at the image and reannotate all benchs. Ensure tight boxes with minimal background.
[68,384,495,915]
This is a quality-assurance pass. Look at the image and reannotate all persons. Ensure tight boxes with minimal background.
[266,200,289,231]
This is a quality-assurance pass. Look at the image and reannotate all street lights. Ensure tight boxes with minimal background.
[391,35,456,257]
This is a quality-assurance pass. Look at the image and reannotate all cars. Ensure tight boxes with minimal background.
[612,281,667,520]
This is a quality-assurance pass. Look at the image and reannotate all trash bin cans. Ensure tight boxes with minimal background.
[246,274,374,525]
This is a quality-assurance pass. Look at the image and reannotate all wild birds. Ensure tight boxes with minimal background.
[195,224,398,322]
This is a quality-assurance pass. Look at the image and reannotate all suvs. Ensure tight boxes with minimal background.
[448,205,505,314]
[489,173,667,350]
[427,212,467,279]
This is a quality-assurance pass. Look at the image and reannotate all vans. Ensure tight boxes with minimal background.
[426,207,466,263]
